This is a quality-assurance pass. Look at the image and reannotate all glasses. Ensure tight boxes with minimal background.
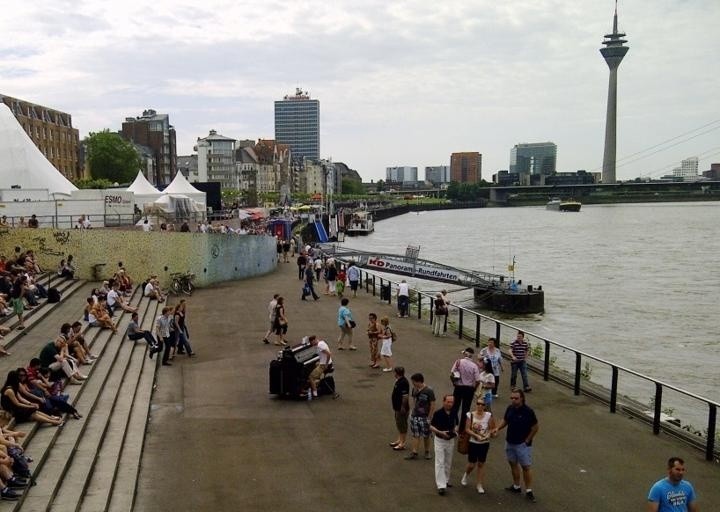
[510,397,519,401]
[477,402,486,406]
[381,320,383,322]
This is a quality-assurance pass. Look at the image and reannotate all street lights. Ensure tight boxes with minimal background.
[222,192,233,219]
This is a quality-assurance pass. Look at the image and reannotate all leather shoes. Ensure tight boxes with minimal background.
[324,292,343,297]
[315,297,320,300]
[439,472,484,496]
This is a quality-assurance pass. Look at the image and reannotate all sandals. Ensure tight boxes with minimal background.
[75,373,88,379]
[70,378,83,384]
[5,303,41,356]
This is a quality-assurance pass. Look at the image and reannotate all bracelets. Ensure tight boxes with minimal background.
[524,441,528,445]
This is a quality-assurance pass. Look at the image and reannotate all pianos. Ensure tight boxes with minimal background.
[269,342,320,397]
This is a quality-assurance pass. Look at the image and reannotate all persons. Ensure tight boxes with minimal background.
[403,373,435,461]
[265,293,288,346]
[338,300,357,350]
[196,209,326,236]
[142,275,166,302]
[1,428,33,501]
[460,398,498,494]
[645,457,699,511]
[366,312,393,372]
[58,255,76,279]
[430,394,458,498]
[78,215,92,229]
[389,367,410,451]
[309,336,332,398]
[1,247,45,329]
[432,289,449,337]
[128,299,194,366]
[277,238,359,299]
[1,322,99,426]
[2,214,38,228]
[84,262,137,331]
[490,390,540,503]
[396,280,408,318]
[134,204,191,233]
[451,329,533,435]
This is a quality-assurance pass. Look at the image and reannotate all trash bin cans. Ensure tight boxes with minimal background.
[96,264,106,280]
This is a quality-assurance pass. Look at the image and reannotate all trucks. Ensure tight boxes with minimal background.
[71,189,141,227]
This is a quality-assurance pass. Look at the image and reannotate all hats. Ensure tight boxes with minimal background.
[466,348,474,354]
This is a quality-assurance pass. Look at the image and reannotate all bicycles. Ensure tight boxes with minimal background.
[169,270,196,296]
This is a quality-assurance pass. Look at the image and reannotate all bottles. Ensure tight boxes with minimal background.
[307,387,312,401]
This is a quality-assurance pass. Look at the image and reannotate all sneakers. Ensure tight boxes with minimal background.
[504,484,521,494]
[493,394,498,398]
[349,346,357,350]
[263,339,288,346]
[82,353,99,365]
[0,474,28,501]
[403,452,418,460]
[424,451,431,460]
[389,441,399,446]
[312,396,320,401]
[149,342,196,366]
[525,490,536,503]
[368,360,392,372]
[338,347,346,350]
[393,444,406,450]
[523,387,532,393]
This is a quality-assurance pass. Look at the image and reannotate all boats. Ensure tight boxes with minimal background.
[559,199,582,213]
[547,198,561,206]
[347,201,374,236]
[473,282,545,313]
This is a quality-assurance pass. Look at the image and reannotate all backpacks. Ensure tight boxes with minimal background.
[385,327,397,341]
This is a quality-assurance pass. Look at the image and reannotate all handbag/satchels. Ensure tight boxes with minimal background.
[451,360,461,382]
[479,359,484,369]
[346,321,356,329]
[279,317,288,326]
[457,411,473,455]
[438,305,447,313]
[302,283,310,296]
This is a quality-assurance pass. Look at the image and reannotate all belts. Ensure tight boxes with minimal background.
[436,435,455,440]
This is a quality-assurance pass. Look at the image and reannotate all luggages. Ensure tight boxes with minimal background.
[47,272,60,303]
